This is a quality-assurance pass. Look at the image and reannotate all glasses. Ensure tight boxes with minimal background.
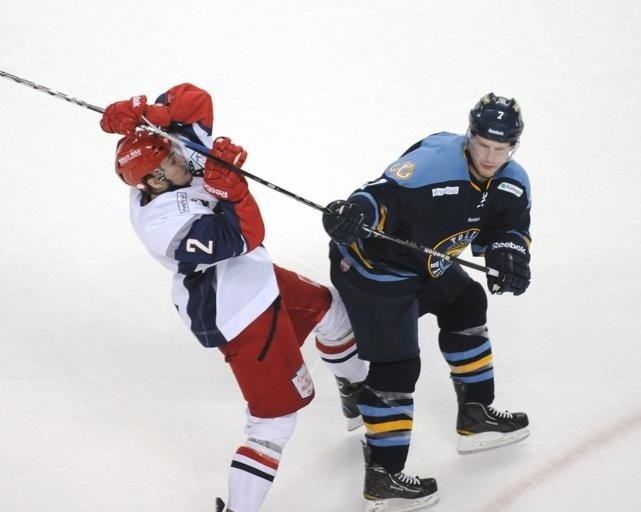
[464,126,521,163]
[154,139,189,182]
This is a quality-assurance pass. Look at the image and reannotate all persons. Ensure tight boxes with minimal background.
[318,91,532,500]
[99,83,369,512]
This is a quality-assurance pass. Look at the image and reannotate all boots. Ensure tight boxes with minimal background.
[336,376,366,419]
[456,394,529,436]
[363,460,438,502]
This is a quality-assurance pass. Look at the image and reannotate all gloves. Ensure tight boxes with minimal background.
[322,200,368,249]
[99,95,171,134]
[484,237,531,296]
[203,138,250,203]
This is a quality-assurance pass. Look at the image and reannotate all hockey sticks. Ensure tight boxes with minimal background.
[142,115,502,278]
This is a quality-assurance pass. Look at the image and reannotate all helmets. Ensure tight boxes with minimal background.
[468,92,525,143]
[114,132,172,193]
